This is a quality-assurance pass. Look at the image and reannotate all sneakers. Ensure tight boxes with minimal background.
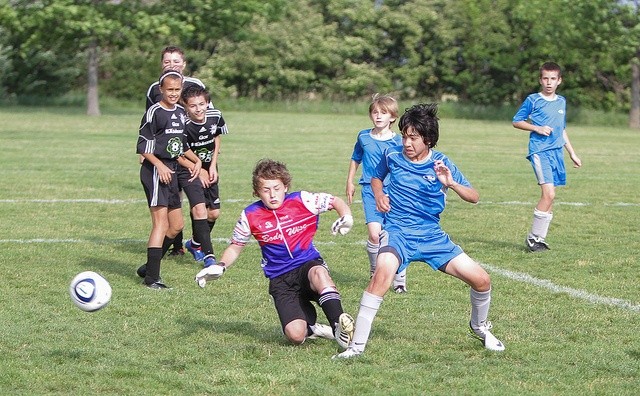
[467,320,505,351]
[309,323,335,338]
[526,233,540,252]
[137,264,146,278]
[335,313,354,349]
[186,239,205,261]
[540,239,551,251]
[168,248,185,256]
[394,285,405,293]
[204,254,217,267]
[148,281,173,291]
[331,341,365,359]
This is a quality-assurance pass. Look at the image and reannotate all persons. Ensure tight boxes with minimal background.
[136,70,203,290]
[345,92,407,294]
[332,105,505,360]
[194,162,356,350]
[142,47,216,111]
[512,62,582,253]
[177,84,229,272]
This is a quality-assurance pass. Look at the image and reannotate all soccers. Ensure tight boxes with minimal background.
[70,271,112,312]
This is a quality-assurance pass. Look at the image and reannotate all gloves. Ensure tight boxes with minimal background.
[331,215,353,235]
[194,261,226,288]
